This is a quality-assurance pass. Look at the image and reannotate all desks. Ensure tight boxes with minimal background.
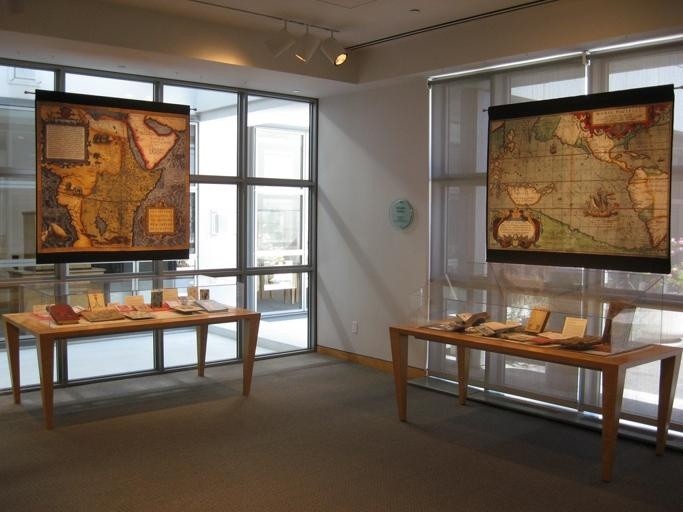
[0,300,260,431]
[388,317,680,484]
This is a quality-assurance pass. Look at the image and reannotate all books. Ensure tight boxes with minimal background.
[421,307,603,352]
[47,287,230,330]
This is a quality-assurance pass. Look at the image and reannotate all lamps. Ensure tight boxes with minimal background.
[263,21,347,66]
